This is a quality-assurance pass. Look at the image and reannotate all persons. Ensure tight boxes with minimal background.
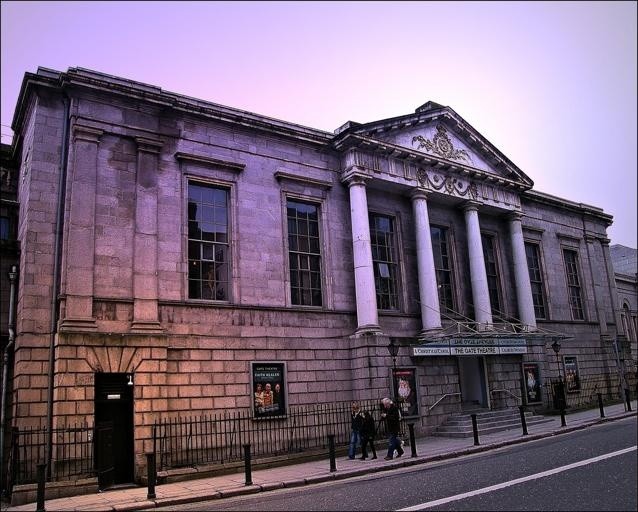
[566,369,577,381]
[347,397,405,461]
[254,383,280,407]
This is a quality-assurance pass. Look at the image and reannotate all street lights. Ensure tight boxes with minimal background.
[551,339,562,381]
[388,340,400,408]
